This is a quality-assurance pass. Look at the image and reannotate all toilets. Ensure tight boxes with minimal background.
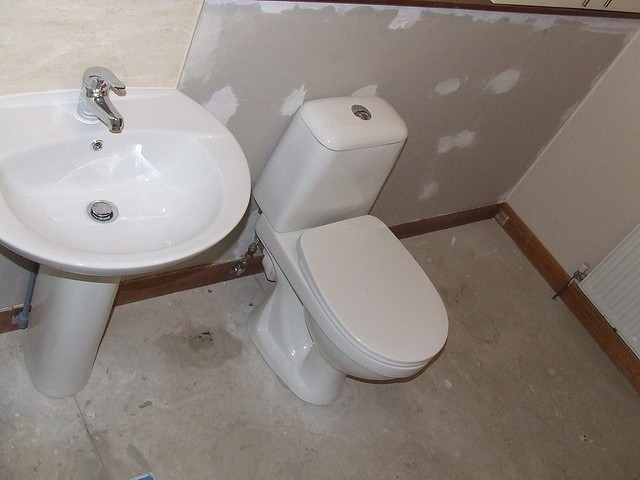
[253,97,450,407]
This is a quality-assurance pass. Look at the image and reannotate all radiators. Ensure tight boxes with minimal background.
[577,221,640,361]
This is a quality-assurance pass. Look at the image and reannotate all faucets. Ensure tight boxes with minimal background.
[77,67,127,134]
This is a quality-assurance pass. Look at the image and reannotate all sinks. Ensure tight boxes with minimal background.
[1,88,253,276]
